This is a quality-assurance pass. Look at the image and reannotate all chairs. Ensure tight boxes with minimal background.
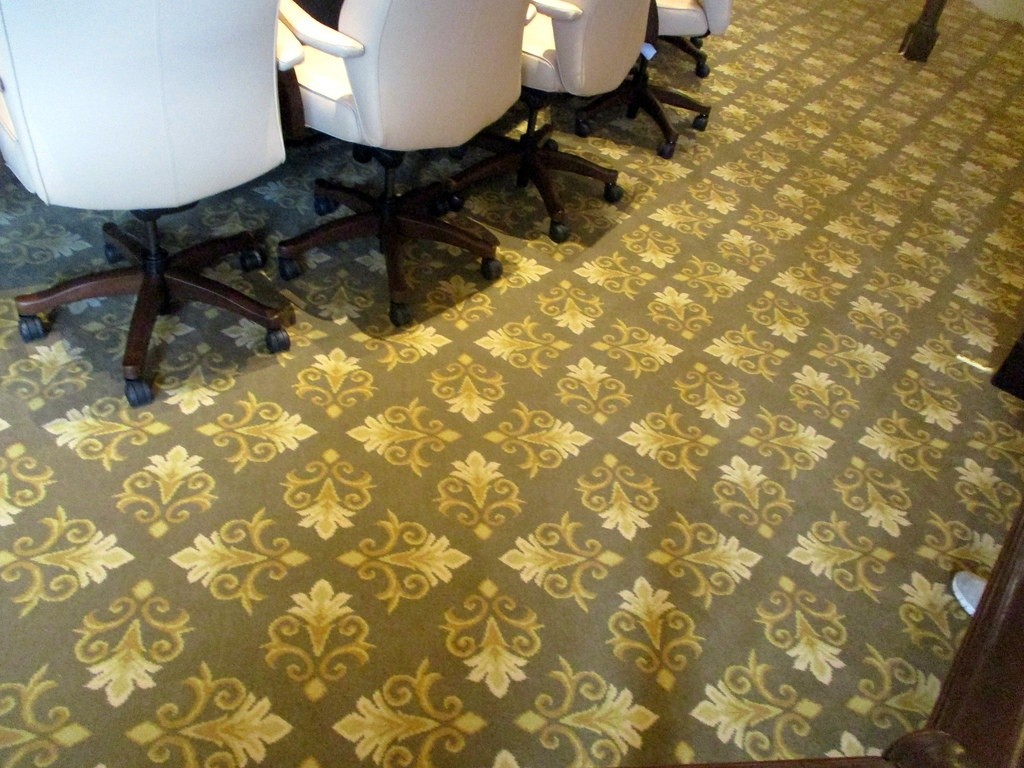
[656,0,733,78]
[572,0,712,161]
[0,0,295,409]
[276,0,584,329]
[447,0,652,243]
